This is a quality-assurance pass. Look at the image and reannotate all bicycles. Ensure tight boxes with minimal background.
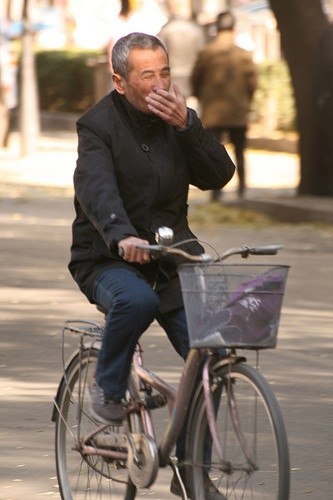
[51,225,291,500]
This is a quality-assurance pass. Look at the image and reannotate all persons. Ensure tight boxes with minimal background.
[68,32,236,500]
[157,0,257,202]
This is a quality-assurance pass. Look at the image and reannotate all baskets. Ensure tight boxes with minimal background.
[177,262,290,350]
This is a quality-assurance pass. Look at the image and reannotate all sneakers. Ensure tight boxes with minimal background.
[86,375,125,425]
[170,472,227,500]
